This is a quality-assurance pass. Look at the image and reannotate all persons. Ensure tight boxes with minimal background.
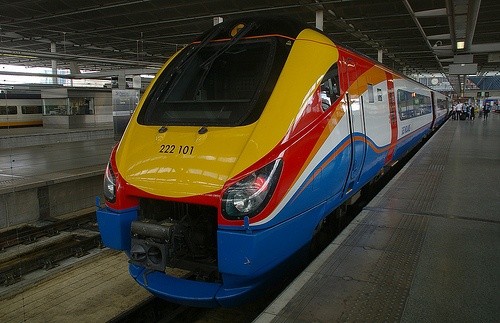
[452,98,493,121]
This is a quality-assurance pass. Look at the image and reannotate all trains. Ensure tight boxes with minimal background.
[95,19,457,308]
[0,93,90,129]
